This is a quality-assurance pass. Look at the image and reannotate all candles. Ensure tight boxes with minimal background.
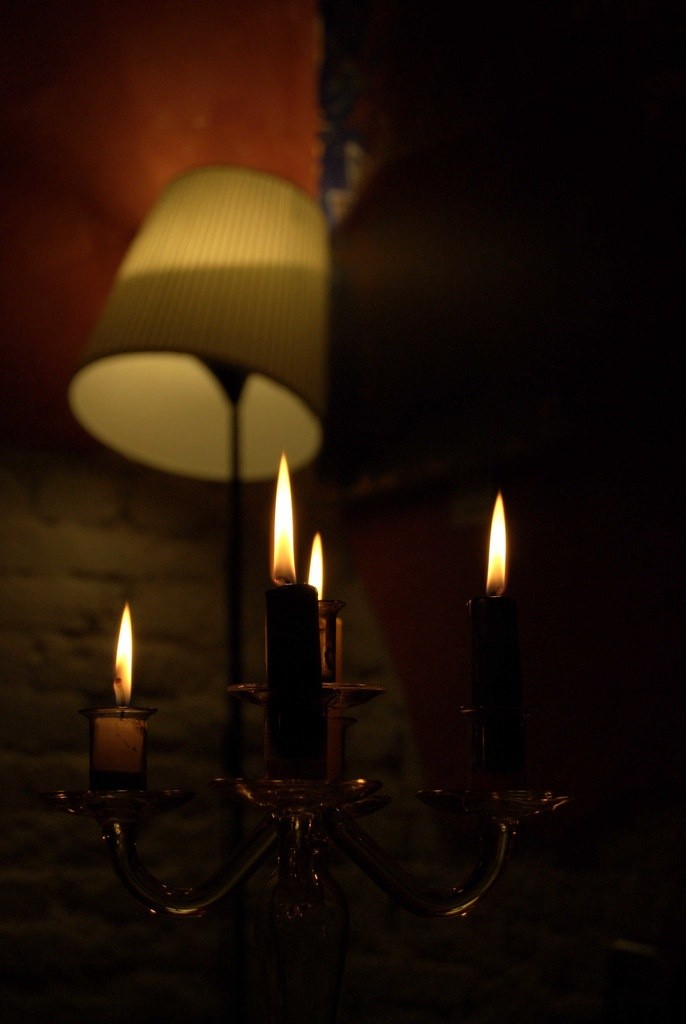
[264,455,325,780]
[469,488,529,788]
[77,596,157,791]
[306,529,346,681]
[327,618,344,782]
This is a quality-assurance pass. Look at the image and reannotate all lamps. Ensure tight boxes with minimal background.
[68,161,333,1024]
